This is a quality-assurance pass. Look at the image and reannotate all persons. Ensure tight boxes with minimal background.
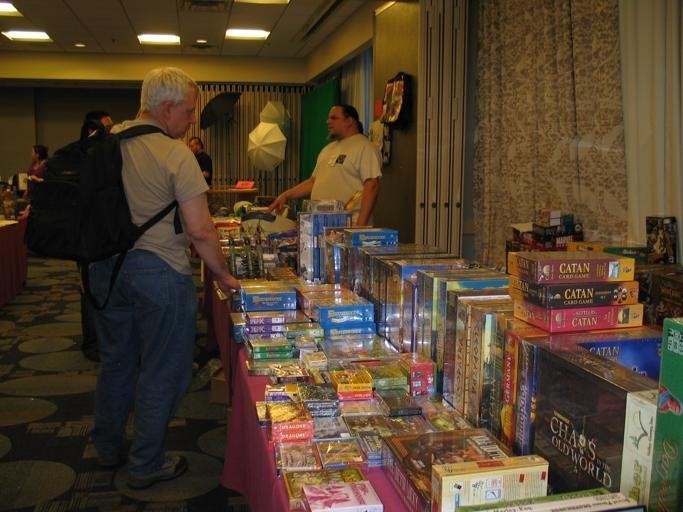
[76,109,115,363]
[187,134,214,187]
[91,64,240,492]
[16,190,32,222]
[21,142,50,196]
[256,103,383,228]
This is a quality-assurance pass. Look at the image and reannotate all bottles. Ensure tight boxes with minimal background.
[226,237,266,279]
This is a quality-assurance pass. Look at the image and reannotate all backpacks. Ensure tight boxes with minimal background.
[22,119,177,263]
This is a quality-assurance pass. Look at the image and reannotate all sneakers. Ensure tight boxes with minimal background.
[127,455,188,490]
[99,428,127,468]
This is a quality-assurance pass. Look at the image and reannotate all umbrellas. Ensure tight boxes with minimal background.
[258,98,291,134]
[199,90,242,131]
[246,120,287,172]
